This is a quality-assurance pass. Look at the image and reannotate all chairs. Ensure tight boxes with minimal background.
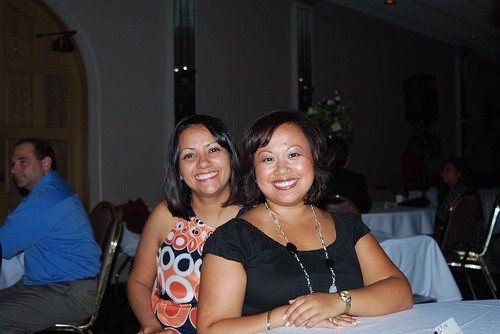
[55,201,123,334]
[447,207,500,301]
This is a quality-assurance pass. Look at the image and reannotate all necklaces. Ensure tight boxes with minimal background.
[264,200,338,295]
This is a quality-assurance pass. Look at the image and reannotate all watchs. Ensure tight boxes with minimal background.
[338,289,352,315]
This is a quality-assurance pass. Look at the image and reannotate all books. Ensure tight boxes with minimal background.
[352,156,373,191]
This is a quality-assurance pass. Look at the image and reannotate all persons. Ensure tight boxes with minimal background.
[0,136,102,334]
[429,156,486,252]
[325,136,373,213]
[127,112,259,334]
[399,136,429,197]
[195,106,415,334]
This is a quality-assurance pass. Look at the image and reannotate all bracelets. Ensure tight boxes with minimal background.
[266,310,272,331]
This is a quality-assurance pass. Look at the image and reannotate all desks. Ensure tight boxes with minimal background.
[115,208,153,283]
[478,189,500,229]
[261,300,500,334]
[371,230,463,303]
[361,206,435,243]
[408,191,437,207]
